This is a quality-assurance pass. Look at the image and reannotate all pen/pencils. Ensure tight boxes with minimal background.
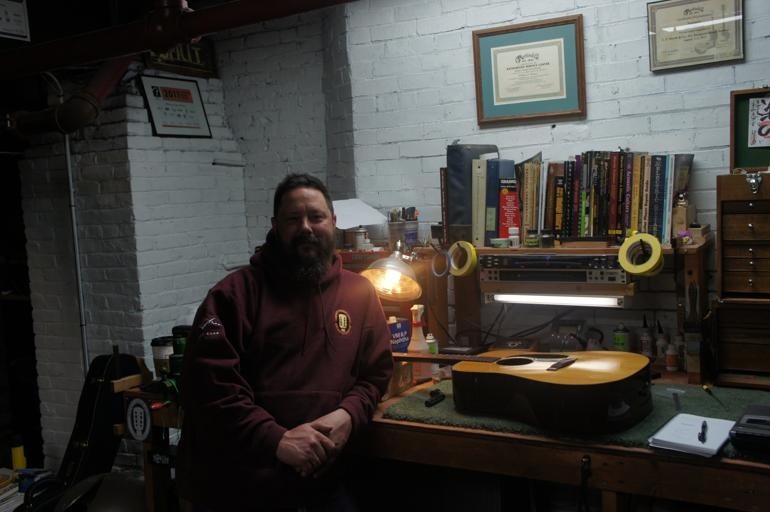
[385,204,419,223]
[696,420,708,442]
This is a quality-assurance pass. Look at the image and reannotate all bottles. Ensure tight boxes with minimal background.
[508,227,520,248]
[664,344,680,371]
[524,229,556,248]
[612,313,668,358]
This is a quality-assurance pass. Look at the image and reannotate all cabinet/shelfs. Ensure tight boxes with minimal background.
[336,246,450,382]
[713,172,770,391]
[453,231,715,386]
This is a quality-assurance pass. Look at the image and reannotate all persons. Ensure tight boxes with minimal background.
[183,173,395,512]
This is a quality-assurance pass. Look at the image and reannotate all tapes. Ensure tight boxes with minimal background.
[617,233,664,278]
[430,251,450,278]
[446,241,478,278]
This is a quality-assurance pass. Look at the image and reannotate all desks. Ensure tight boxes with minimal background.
[354,376,770,512]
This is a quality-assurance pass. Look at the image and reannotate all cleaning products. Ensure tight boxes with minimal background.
[408,305,431,381]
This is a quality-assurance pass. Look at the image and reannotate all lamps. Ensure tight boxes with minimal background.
[356,239,422,303]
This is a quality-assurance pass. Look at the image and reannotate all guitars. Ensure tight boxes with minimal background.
[393,349,653,434]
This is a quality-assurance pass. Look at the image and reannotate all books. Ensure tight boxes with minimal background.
[499,150,693,248]
[647,412,736,458]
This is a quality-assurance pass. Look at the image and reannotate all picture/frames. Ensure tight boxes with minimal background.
[729,86,770,173]
[472,14,586,124]
